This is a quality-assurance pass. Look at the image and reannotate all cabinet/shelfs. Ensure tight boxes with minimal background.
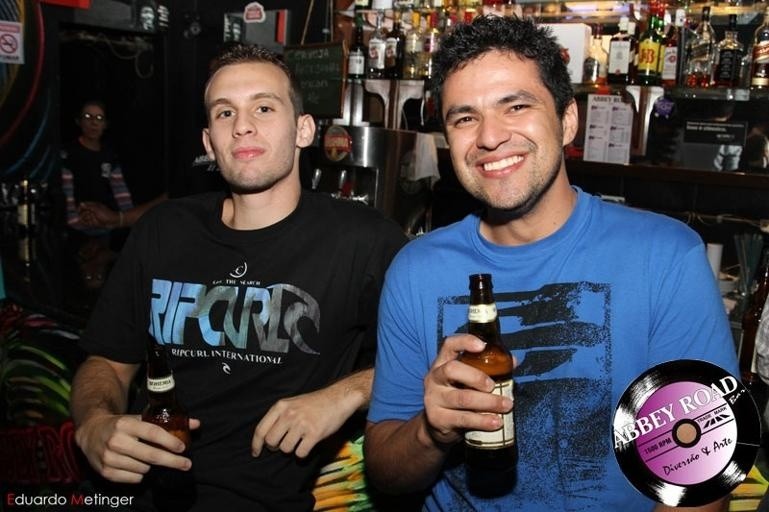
[332,1,769,104]
[427,146,768,377]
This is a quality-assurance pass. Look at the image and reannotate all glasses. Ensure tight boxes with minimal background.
[80,113,105,122]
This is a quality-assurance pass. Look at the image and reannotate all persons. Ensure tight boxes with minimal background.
[362,14,744,512]
[59,100,135,229]
[76,41,313,230]
[69,45,414,512]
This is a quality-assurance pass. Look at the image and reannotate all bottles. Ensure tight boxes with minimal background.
[15,177,39,234]
[343,1,768,79]
[461,271,520,500]
[17,234,36,266]
[141,345,192,451]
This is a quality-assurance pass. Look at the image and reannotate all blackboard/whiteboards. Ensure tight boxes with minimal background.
[281,38,349,119]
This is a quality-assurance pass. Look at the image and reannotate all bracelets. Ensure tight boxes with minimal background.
[118,210,124,229]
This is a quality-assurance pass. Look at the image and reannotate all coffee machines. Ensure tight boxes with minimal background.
[311,124,433,244]
